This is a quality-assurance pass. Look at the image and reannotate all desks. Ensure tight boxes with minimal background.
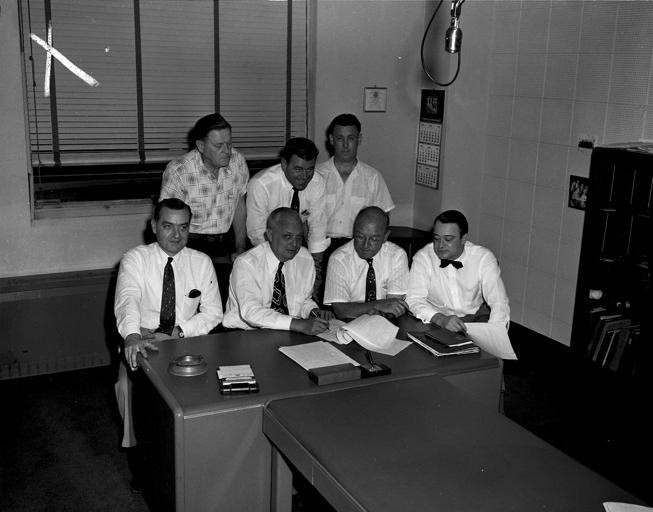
[248,366,647,512]
[381,224,432,264]
[120,304,510,512]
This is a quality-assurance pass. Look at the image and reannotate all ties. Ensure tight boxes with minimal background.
[291,187,300,214]
[154,256,176,337]
[270,260,289,315]
[365,258,376,303]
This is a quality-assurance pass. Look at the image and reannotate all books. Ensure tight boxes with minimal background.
[406,326,481,360]
[316,312,414,356]
[215,364,261,396]
[278,340,360,371]
[578,307,644,373]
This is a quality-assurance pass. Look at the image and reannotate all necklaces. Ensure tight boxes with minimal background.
[333,159,356,178]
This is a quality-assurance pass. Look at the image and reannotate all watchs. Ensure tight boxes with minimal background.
[175,326,183,338]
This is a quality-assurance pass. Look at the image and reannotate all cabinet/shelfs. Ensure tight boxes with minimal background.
[549,138,652,427]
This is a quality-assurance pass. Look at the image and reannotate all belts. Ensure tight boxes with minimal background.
[189,233,230,243]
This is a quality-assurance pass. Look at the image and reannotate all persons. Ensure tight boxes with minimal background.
[155,113,250,335]
[405,208,511,336]
[110,197,225,495]
[222,205,336,337]
[244,135,334,311]
[314,112,399,312]
[320,204,408,321]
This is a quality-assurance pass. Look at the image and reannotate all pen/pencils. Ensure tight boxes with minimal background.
[367,350,377,369]
[312,311,330,331]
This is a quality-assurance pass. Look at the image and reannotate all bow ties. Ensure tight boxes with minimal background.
[438,259,464,269]
